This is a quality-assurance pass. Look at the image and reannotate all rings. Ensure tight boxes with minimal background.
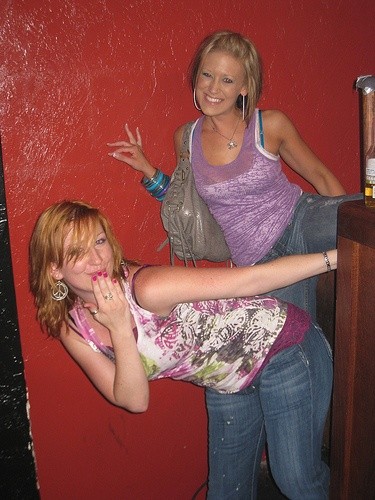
[104,292,113,300]
[90,309,99,315]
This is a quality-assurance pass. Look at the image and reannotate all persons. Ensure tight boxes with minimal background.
[28,198,338,500]
[105,30,365,324]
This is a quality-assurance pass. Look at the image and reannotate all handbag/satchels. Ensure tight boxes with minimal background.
[158,120,230,267]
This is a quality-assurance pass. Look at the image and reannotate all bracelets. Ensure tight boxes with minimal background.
[143,169,171,202]
[322,251,331,273]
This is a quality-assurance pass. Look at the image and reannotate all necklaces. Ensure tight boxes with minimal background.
[212,115,242,150]
